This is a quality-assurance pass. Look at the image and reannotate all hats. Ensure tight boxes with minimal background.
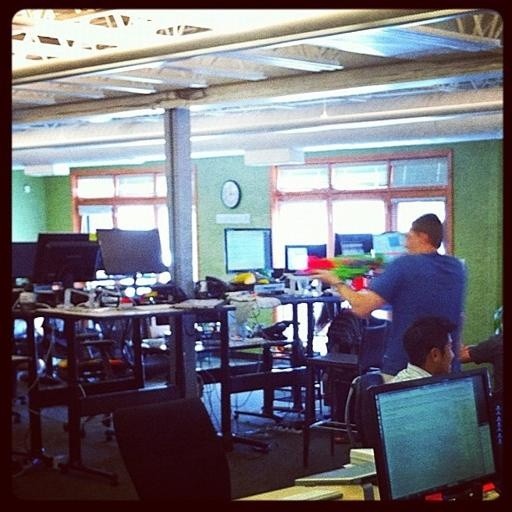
[412,214,442,249]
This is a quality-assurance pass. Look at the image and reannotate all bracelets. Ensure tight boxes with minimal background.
[333,282,345,288]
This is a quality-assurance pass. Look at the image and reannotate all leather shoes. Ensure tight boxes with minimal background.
[335,433,361,443]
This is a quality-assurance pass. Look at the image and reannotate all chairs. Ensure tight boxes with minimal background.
[104,398,249,500]
[299,321,387,467]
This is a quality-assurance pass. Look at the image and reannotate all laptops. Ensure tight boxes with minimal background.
[294,461,378,485]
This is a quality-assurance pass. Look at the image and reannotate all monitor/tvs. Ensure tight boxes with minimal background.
[367,367,505,505]
[95,228,164,287]
[224,227,272,273]
[334,233,373,258]
[12,242,38,287]
[32,233,101,292]
[285,245,326,274]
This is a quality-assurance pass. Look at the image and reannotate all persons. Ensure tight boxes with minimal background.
[380,315,455,387]
[319,214,465,441]
[457,306,504,421]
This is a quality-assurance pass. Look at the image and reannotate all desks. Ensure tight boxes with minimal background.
[11,285,347,471]
